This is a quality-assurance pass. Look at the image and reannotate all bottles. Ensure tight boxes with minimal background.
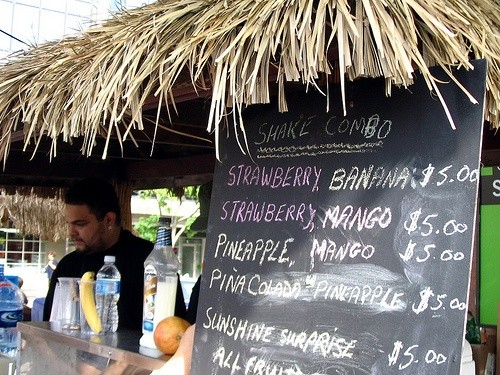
[138,216,181,347]
[0,263,25,358]
[93,255,121,333]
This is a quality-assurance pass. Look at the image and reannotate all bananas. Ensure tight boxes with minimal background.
[81,272,104,333]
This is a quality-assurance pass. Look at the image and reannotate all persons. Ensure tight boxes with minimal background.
[43,176,186,329]
[45,251,57,286]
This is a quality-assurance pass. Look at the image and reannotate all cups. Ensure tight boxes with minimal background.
[59,277,82,330]
[76,280,112,337]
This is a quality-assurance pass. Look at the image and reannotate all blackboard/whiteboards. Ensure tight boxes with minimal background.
[186,57,486,375]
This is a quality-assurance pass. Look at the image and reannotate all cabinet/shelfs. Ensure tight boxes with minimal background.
[16,321,176,375]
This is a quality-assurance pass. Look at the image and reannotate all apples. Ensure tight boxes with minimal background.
[154,317,191,355]
